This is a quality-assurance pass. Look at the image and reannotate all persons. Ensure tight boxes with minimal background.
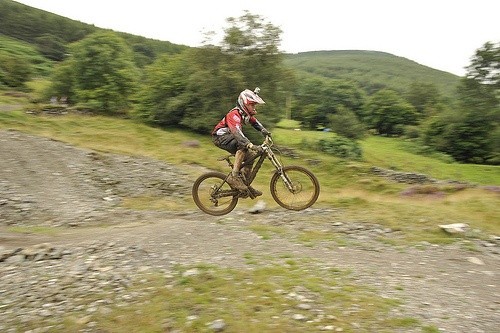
[211,87,271,196]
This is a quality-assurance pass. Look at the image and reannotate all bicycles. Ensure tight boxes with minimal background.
[192,133,319,216]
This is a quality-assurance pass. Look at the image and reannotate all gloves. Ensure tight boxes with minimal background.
[261,128,272,138]
[251,145,264,152]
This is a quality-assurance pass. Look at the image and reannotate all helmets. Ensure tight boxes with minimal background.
[237,88,266,119]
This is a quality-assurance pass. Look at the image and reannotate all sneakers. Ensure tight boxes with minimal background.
[248,186,263,196]
[226,171,249,191]
